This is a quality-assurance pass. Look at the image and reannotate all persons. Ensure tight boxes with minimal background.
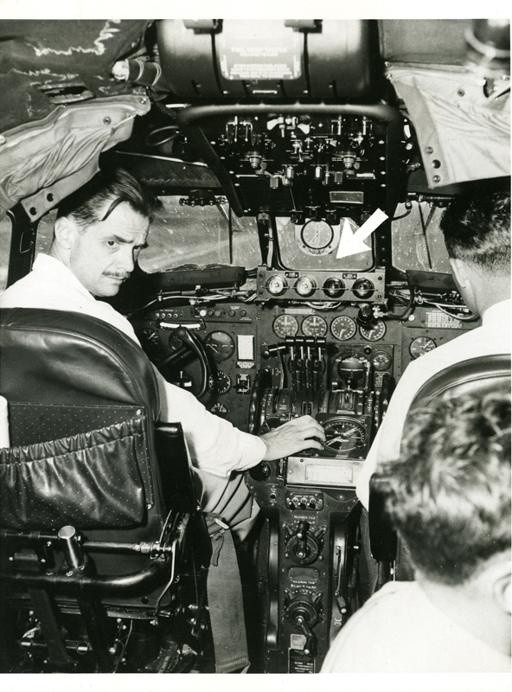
[354,183,511,512]
[318,388,512,673]
[1,171,327,541]
